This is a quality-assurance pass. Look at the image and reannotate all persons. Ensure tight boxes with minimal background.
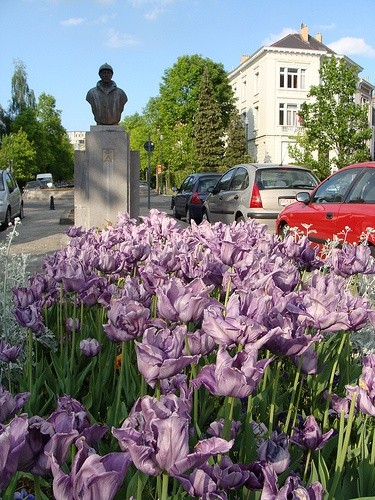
[85,63,128,125]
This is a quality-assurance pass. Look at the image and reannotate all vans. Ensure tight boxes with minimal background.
[36,172,54,189]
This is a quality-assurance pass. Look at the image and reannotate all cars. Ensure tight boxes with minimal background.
[0,170,24,229]
[22,180,47,194]
[170,173,223,223]
[199,163,322,234]
[275,161,375,258]
